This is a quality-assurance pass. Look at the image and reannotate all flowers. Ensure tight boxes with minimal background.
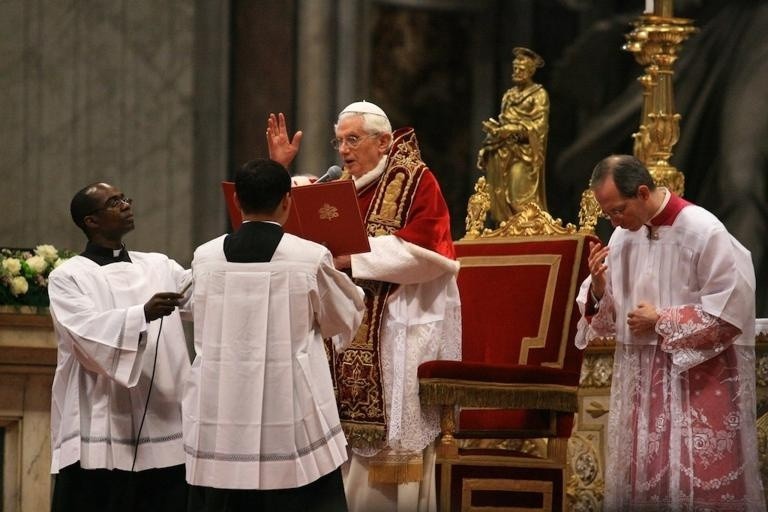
[0,244,81,299]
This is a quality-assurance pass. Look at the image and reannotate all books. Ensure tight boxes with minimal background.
[216,179,371,258]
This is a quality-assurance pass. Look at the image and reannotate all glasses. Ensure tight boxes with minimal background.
[603,200,631,218]
[330,130,381,150]
[84,196,134,217]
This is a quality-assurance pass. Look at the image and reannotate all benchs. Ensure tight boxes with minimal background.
[418,232,599,512]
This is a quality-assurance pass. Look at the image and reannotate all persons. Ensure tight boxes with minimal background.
[474,46,551,230]
[264,100,463,511]
[572,152,768,511]
[181,156,368,512]
[46,181,193,510]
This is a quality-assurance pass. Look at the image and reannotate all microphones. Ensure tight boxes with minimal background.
[313,165,343,184]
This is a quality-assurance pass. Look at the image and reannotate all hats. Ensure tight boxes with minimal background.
[338,99,390,121]
